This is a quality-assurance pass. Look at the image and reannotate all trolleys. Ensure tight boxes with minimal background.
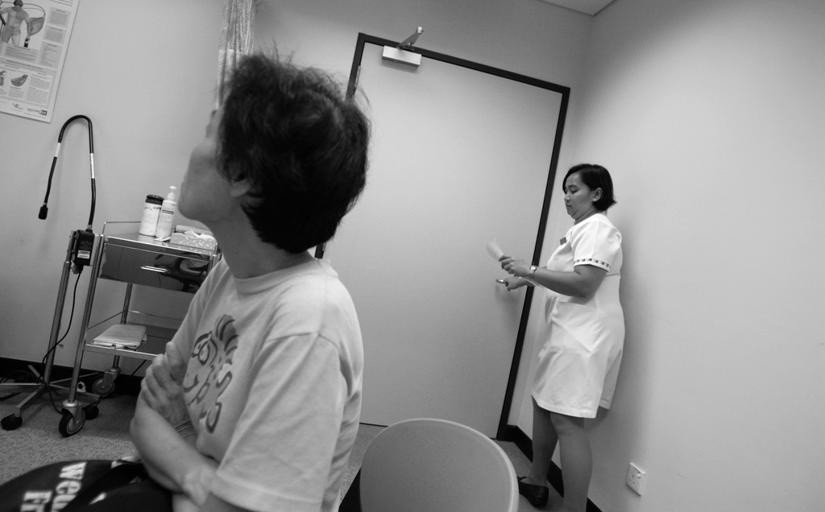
[56,219,223,438]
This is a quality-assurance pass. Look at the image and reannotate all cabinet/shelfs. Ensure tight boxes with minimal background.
[59,220,220,439]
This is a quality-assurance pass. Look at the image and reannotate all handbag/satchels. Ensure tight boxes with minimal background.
[1,460,172,512]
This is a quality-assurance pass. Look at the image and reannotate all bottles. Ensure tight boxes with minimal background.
[138,194,163,237]
[486,242,508,262]
[157,185,176,240]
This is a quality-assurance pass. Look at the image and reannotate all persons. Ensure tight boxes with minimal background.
[0,0,30,46]
[130,54,368,512]
[498,164,626,512]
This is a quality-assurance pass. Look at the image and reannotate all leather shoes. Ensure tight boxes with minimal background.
[517,476,549,508]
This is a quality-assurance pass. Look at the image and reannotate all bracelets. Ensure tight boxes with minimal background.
[529,265,537,280]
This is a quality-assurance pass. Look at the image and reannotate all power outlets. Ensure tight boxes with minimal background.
[626,462,646,496]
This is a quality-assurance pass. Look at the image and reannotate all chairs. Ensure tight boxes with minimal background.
[340,417,520,512]
